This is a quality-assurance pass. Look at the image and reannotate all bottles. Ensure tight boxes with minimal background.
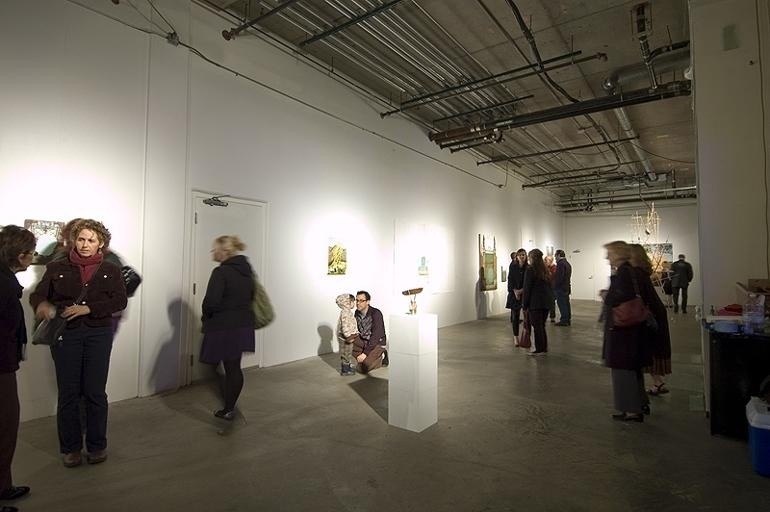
[708,319,739,333]
[741,292,766,335]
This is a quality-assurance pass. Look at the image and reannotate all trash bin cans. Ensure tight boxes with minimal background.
[746,400,770,477]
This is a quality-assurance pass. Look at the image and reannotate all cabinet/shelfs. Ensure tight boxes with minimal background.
[706,320,770,443]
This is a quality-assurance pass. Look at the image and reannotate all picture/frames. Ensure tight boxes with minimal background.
[478,233,496,255]
[479,252,498,291]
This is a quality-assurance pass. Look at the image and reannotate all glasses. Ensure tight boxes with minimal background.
[356,299,367,303]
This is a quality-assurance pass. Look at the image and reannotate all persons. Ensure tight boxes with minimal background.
[602,240,657,423]
[627,244,672,395]
[29,219,143,472]
[1,224,36,512]
[336,294,360,376]
[669,254,693,313]
[352,291,389,372]
[661,261,673,308]
[200,236,255,436]
[505,249,571,356]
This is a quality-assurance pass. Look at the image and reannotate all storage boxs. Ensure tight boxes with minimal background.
[743,394,770,477]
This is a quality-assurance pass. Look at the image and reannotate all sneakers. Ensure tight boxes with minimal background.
[550,319,571,327]
[86,447,110,464]
[213,408,234,420]
[611,403,652,423]
[513,337,520,347]
[512,317,523,324]
[217,412,249,438]
[340,368,356,376]
[61,447,83,468]
[1,506,19,511]
[645,379,669,396]
[3,484,31,502]
[380,348,389,367]
[528,348,548,358]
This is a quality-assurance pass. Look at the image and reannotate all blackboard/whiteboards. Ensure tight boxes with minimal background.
[483,252,497,289]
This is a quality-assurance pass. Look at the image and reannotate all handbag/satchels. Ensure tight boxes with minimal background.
[27,299,74,346]
[603,265,655,328]
[517,309,533,348]
[250,267,276,333]
